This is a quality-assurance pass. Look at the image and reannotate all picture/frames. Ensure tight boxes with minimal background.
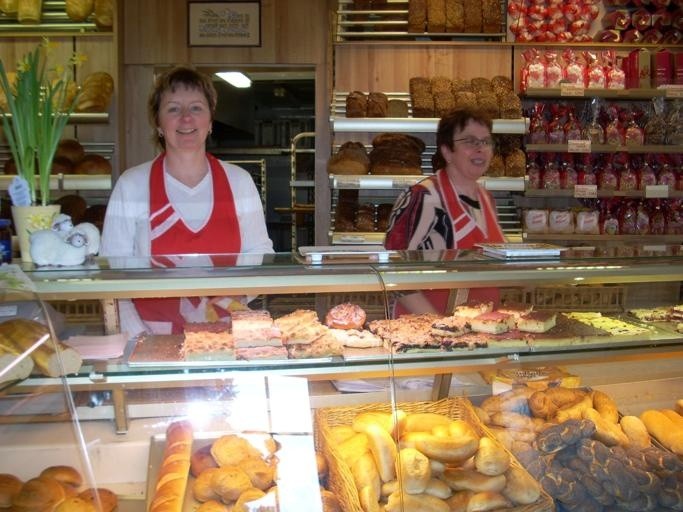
[186,1,262,47]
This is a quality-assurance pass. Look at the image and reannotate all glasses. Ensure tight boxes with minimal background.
[450,135,497,150]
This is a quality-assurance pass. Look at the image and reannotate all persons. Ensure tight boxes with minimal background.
[100,66,274,337]
[383,106,508,320]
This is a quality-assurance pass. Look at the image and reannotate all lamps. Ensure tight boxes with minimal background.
[214,68,256,90]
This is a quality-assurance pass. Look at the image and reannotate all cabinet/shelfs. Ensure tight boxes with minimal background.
[332,1,682,258]
[3,1,122,194]
[2,258,683,512]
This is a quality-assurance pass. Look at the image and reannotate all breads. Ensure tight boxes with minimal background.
[233,489,267,512]
[346,90,366,116]
[75,154,113,174]
[378,204,394,230]
[1,0,18,24]
[395,448,430,496]
[386,99,407,116]
[77,71,114,114]
[407,0,427,33]
[41,467,83,488]
[80,489,116,511]
[329,424,371,461]
[239,460,272,490]
[425,456,508,498]
[507,151,525,174]
[535,418,596,452]
[335,187,359,232]
[642,399,682,454]
[409,77,430,94]
[456,92,478,117]
[0,71,18,112]
[329,142,370,173]
[366,426,396,482]
[195,501,226,512]
[473,387,544,450]
[394,411,479,464]
[529,389,593,424]
[17,0,42,23]
[213,465,254,501]
[385,491,451,511]
[594,390,619,425]
[1,320,82,381]
[500,91,522,118]
[211,434,262,470]
[130,334,186,364]
[16,478,64,512]
[349,460,383,512]
[55,497,100,512]
[451,75,473,91]
[191,444,220,476]
[513,440,683,511]
[268,485,278,510]
[0,475,23,508]
[384,479,451,499]
[65,1,93,23]
[48,76,76,112]
[502,466,540,504]
[446,0,463,33]
[474,437,510,475]
[478,94,501,118]
[149,421,193,512]
[482,0,502,34]
[367,91,387,117]
[85,205,107,234]
[491,75,512,93]
[467,491,515,512]
[95,0,115,30]
[485,149,506,177]
[430,75,451,91]
[3,139,83,175]
[464,0,484,33]
[582,409,629,445]
[352,411,395,433]
[371,133,426,177]
[412,93,434,116]
[356,201,374,230]
[428,1,446,34]
[192,469,223,503]
[437,92,455,116]
[54,196,83,220]
[620,417,650,451]
[472,76,492,94]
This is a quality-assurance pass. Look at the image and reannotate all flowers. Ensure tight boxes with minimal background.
[0,34,95,203]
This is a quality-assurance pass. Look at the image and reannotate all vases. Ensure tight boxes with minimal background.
[11,203,62,273]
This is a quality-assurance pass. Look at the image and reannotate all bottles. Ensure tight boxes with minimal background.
[0,219,12,265]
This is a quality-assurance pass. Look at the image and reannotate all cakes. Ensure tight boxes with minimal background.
[491,368,582,396]
[515,308,611,345]
[496,300,533,320]
[274,310,342,359]
[183,321,234,354]
[325,303,364,329]
[487,332,528,352]
[367,313,487,350]
[231,309,288,361]
[454,302,493,318]
[473,313,512,333]
[328,328,383,347]
